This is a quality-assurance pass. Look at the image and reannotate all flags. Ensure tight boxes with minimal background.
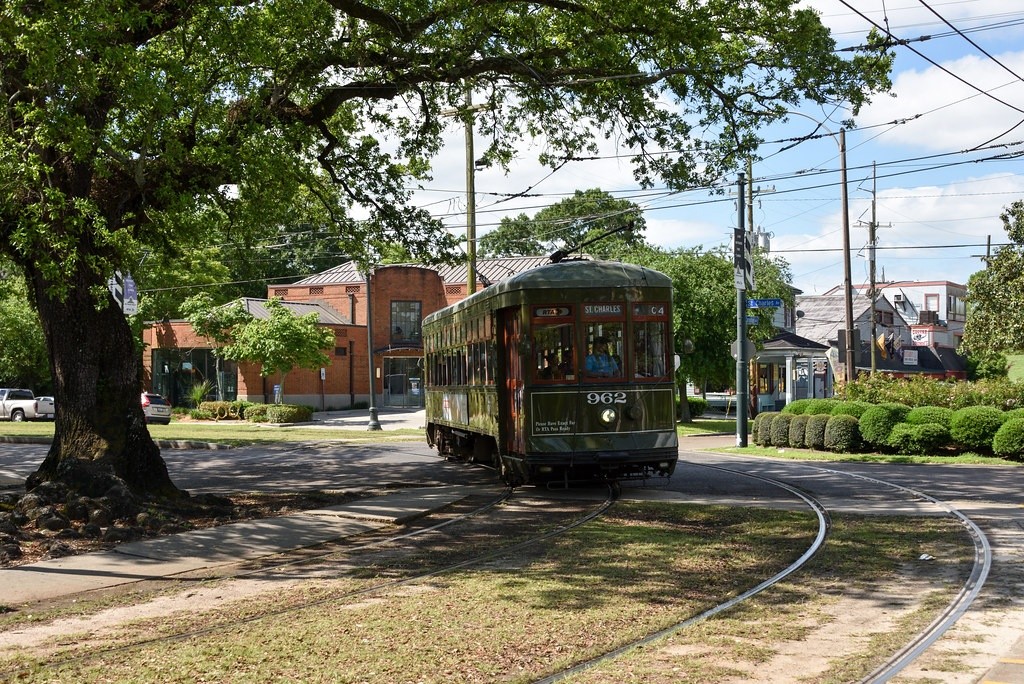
[876,333,887,360]
[894,337,903,358]
[886,333,895,361]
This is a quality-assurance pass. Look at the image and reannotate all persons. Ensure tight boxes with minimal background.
[634,328,653,376]
[586,337,621,377]
[544,350,572,378]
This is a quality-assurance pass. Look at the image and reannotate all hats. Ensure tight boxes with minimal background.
[593,336,612,344]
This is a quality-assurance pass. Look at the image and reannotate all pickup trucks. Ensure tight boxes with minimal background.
[0,388,54,422]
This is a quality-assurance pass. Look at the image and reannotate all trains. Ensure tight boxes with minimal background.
[422,262,679,487]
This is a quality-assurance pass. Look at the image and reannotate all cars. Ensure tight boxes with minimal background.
[139,393,172,425]
